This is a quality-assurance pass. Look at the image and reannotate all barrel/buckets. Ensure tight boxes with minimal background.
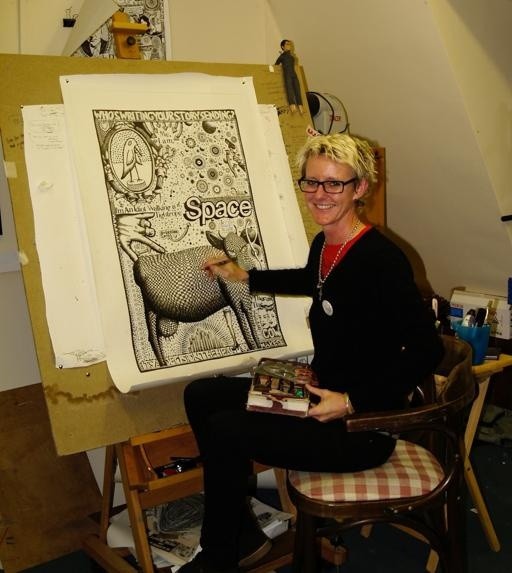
[451,320,492,367]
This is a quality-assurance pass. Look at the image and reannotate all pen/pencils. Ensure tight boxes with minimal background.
[460,307,489,327]
[201,255,238,271]
[483,355,499,360]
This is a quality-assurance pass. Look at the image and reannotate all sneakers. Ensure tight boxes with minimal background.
[175,530,272,573]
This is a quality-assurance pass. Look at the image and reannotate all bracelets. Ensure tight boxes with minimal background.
[342,392,355,415]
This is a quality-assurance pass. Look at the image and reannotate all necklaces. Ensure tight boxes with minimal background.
[316,216,363,302]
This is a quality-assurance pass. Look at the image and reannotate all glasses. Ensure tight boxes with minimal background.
[298,177,356,194]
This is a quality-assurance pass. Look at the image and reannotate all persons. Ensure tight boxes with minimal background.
[171,127,444,570]
[274,38,304,116]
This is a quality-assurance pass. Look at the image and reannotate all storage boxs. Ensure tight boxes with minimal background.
[448,285,512,340]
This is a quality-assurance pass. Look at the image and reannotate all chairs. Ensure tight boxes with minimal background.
[287,332,479,573]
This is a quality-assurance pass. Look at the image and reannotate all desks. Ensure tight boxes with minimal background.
[451,353,512,553]
